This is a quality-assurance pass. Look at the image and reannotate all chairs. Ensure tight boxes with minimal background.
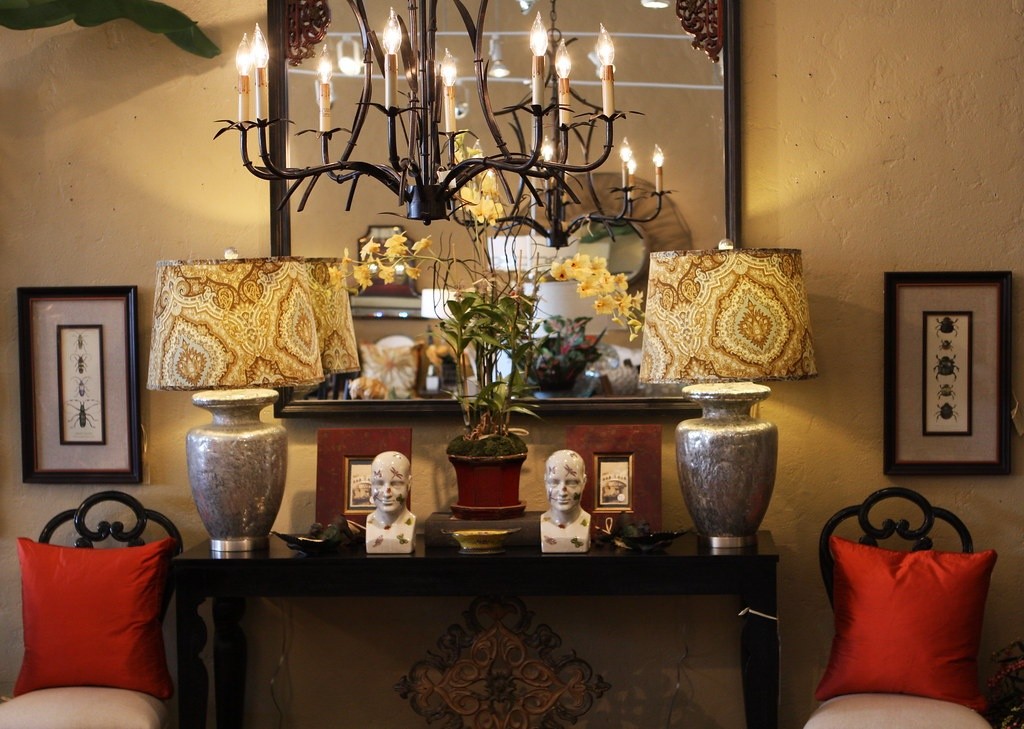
[0,490,183,729]
[801,486,993,729]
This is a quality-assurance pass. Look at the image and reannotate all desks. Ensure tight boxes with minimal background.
[171,531,781,729]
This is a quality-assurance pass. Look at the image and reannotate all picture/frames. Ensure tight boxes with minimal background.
[315,427,412,529]
[884,271,1013,474]
[17,286,143,485]
[356,225,421,298]
[564,424,662,541]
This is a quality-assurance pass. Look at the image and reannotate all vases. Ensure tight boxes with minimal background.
[445,435,530,520]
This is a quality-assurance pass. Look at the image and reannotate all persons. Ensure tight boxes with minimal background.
[365,451,417,555]
[540,450,592,554]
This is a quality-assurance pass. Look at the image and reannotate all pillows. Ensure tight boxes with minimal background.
[813,534,996,711]
[13,537,176,701]
[349,342,422,400]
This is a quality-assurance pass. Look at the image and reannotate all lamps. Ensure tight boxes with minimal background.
[304,257,360,377]
[147,249,325,552]
[434,0,680,248]
[638,239,820,548]
[211,0,645,221]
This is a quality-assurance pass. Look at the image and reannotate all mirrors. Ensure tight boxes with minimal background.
[266,0,740,321]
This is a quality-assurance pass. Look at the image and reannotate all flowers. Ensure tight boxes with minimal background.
[344,138,647,399]
[341,130,649,439]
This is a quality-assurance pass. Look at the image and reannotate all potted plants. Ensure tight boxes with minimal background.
[518,314,607,398]
